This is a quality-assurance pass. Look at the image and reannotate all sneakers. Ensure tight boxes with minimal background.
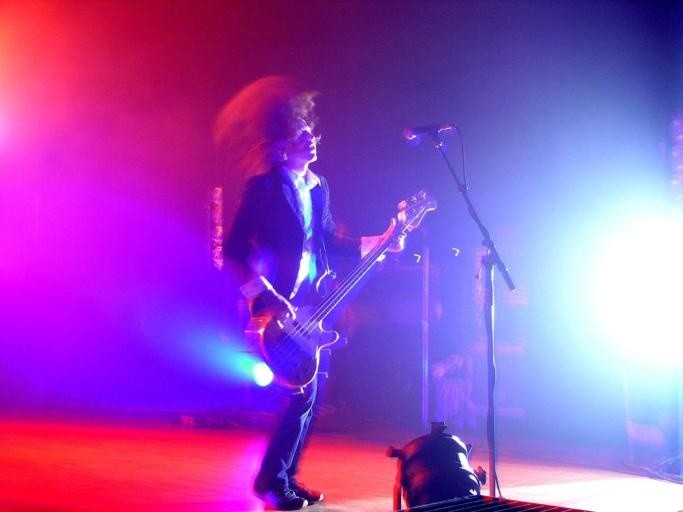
[251,473,309,512]
[288,475,324,506]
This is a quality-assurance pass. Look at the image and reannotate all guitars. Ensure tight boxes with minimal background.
[244,190,438,389]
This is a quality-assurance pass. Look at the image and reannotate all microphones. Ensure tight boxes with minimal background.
[401,121,457,145]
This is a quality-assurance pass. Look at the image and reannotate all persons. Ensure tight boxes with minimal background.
[221,94,413,510]
